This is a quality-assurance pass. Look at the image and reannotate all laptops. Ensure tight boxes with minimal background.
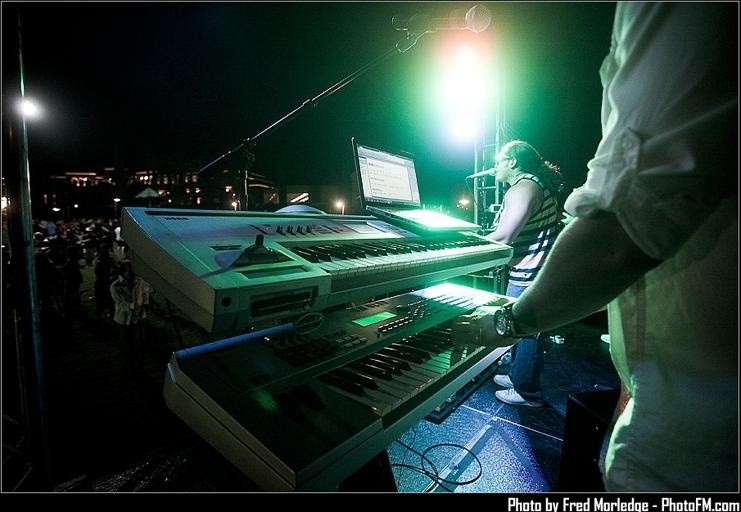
[351,137,483,232]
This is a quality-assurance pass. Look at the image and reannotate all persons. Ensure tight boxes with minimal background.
[30,216,134,323]
[109,261,152,376]
[451,2,738,493]
[481,138,563,408]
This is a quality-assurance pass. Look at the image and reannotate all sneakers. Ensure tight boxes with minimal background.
[494,374,514,388]
[601,334,610,343]
[495,389,545,408]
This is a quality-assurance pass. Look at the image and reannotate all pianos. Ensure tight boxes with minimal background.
[163,281,520,493]
[120,205,515,332]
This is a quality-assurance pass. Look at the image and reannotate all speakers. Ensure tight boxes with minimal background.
[558,388,620,493]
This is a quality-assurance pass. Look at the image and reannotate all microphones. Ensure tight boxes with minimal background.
[466,168,497,179]
[392,4,491,33]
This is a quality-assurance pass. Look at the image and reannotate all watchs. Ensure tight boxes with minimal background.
[493,300,537,341]
